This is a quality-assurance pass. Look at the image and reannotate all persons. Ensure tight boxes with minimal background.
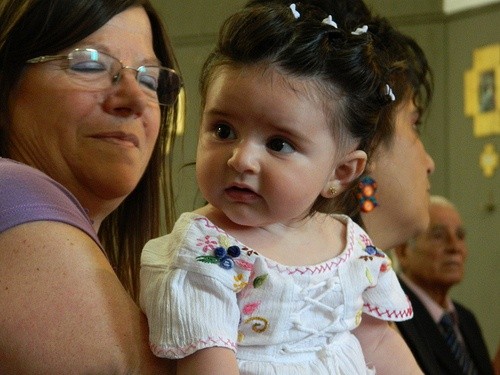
[387,195,495,375]
[334,33,436,254]
[1,0,185,375]
[140,0,422,375]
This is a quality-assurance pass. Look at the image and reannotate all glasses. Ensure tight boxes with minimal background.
[19,48,185,106]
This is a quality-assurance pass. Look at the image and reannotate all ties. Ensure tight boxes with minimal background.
[441,312,474,375]
